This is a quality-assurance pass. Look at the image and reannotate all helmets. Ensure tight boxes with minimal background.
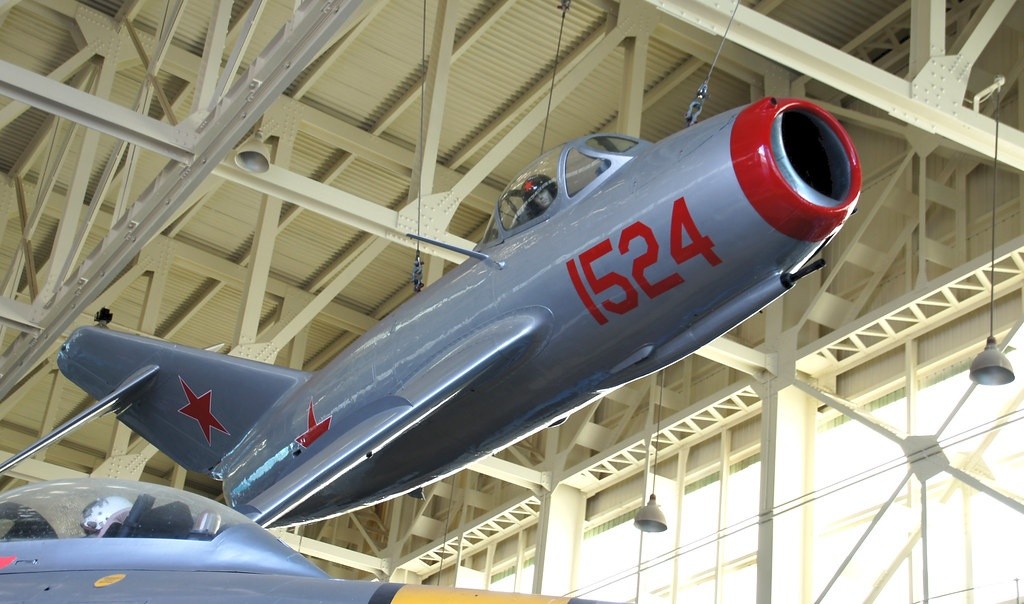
[520,173,556,215]
[80,495,133,535]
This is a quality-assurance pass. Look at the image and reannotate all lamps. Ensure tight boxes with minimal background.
[235,128,271,173]
[634,370,667,532]
[968,78,1016,384]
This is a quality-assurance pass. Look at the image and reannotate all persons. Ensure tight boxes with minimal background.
[523,174,558,219]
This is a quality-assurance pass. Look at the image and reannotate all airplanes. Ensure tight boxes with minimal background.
[0,476,636,604]
[0,96,864,537]
